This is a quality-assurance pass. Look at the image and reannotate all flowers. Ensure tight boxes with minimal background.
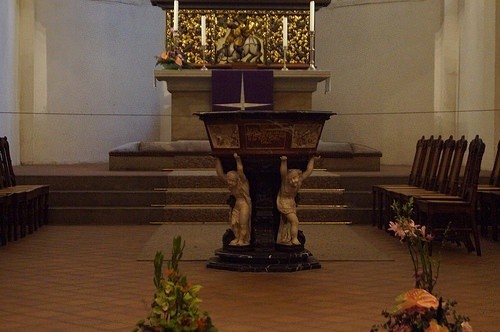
[134,234,217,332]
[370,197,472,332]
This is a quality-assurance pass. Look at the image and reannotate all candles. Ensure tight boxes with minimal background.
[283,17,287,46]
[310,1,315,31]
[173,0,179,30]
[200,15,207,45]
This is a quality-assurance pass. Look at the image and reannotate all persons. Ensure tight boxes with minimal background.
[214,152,251,246]
[277,156,321,245]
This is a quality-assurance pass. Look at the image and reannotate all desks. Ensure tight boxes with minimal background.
[192,110,337,273]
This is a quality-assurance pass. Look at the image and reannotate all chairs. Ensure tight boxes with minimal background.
[371,134,500,256]
[0,136,49,246]
